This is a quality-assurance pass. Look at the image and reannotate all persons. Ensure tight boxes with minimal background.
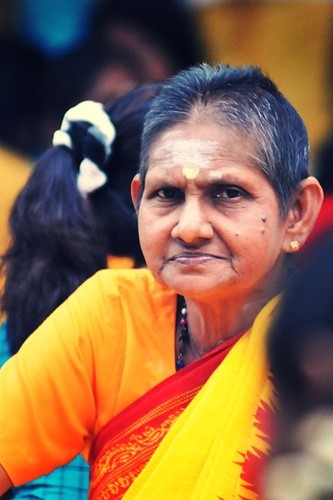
[0,83,164,500]
[0,63,325,500]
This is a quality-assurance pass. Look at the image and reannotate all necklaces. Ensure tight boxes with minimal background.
[177,302,189,368]
